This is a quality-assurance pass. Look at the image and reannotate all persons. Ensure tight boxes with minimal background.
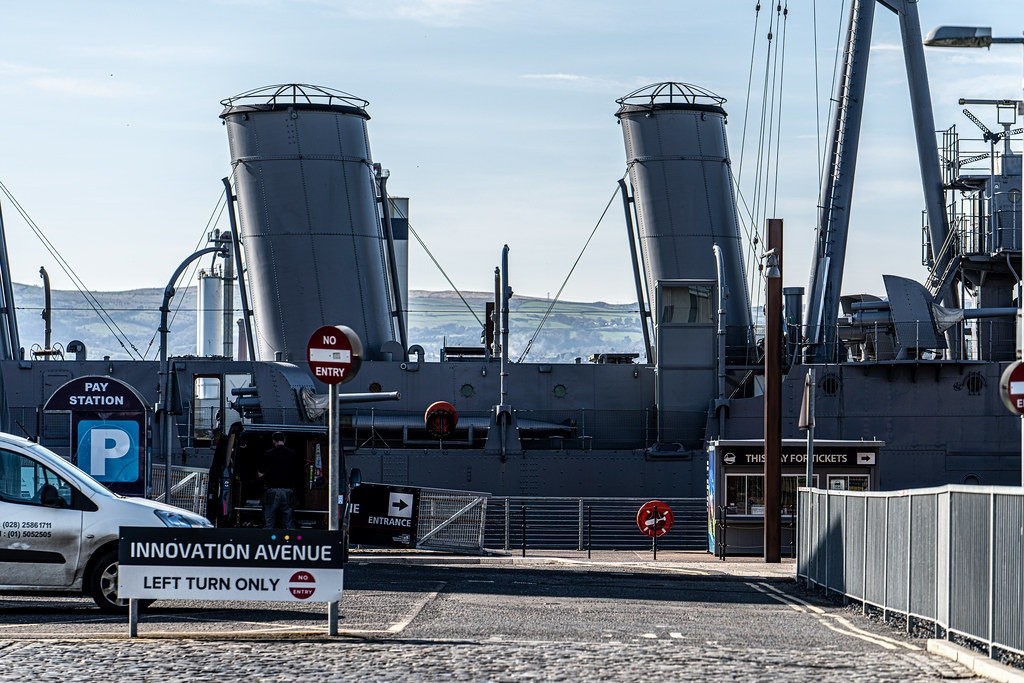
[255,431,298,530]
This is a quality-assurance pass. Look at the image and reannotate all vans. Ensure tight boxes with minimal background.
[207,423,362,525]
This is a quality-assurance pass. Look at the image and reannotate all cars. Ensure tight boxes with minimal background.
[0,431,216,615]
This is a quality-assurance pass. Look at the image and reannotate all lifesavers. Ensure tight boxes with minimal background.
[424,400,459,440]
[636,499,674,538]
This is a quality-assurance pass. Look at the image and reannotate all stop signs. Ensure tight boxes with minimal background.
[1000,359,1023,416]
[289,572,317,600]
[305,325,365,385]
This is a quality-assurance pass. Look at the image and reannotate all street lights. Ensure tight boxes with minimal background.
[761,218,783,563]
